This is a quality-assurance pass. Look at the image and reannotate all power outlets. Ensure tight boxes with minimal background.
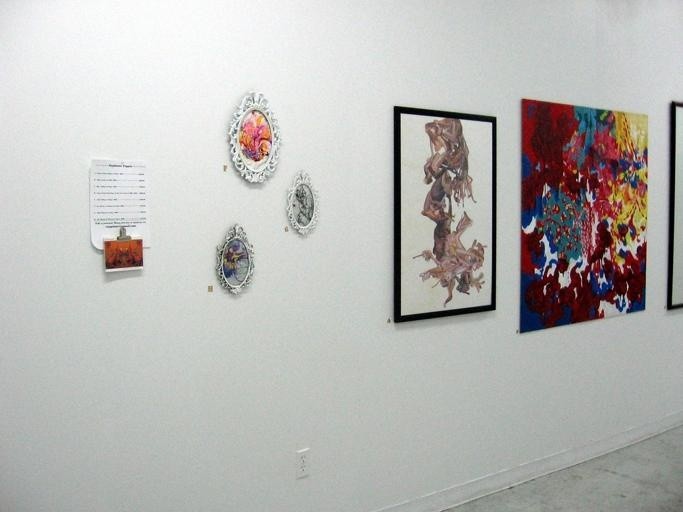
[295,447,313,480]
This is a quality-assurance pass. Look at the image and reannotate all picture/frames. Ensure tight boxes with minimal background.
[664,100,683,310]
[284,169,322,239]
[391,104,496,322]
[226,89,283,185]
[213,223,256,295]
